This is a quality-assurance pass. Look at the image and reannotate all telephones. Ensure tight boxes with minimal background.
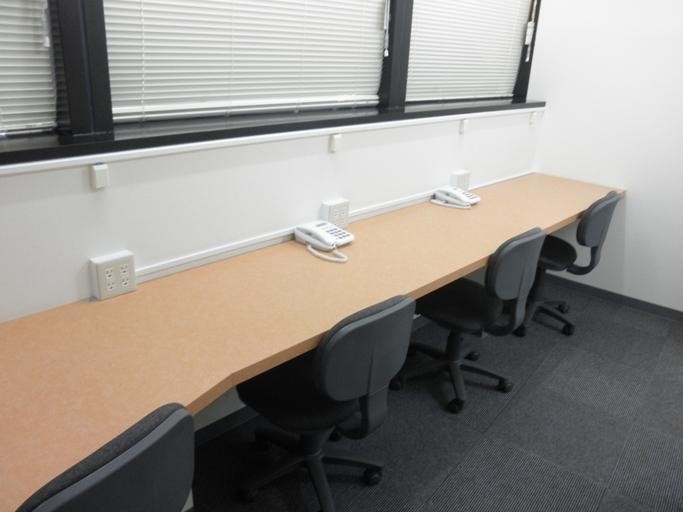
[294,219,354,252]
[434,186,480,206]
